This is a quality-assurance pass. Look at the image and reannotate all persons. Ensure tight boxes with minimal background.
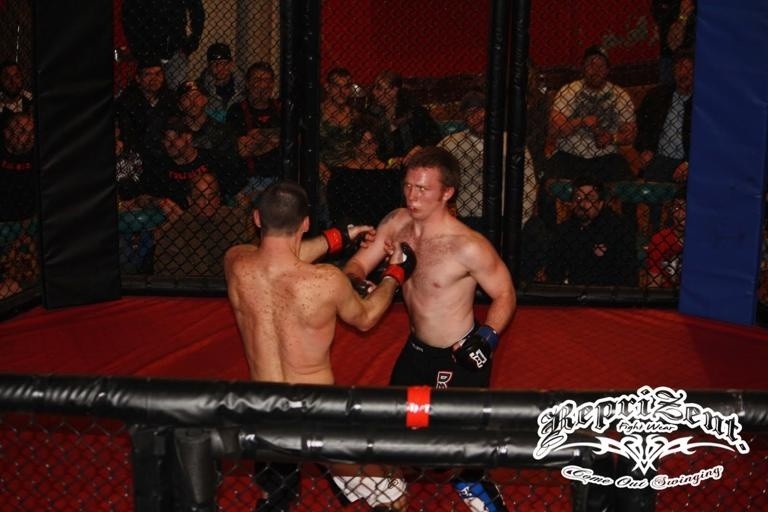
[343,146,520,512]
[223,186,418,512]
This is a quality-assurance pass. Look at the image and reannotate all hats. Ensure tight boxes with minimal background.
[583,44,610,62]
[206,43,232,61]
